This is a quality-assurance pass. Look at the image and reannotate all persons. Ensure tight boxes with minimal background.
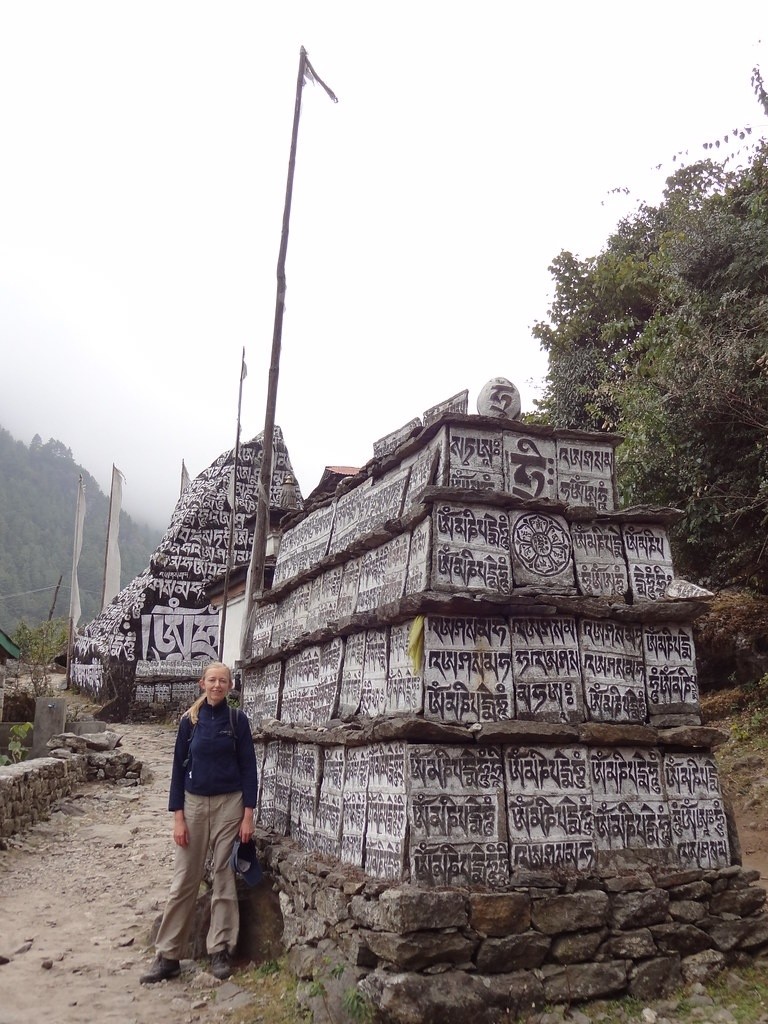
[140,662,258,984]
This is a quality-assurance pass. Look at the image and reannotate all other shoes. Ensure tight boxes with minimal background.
[208,950,233,980]
[139,951,182,985]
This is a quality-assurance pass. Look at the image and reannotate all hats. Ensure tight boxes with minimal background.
[229,835,262,887]
